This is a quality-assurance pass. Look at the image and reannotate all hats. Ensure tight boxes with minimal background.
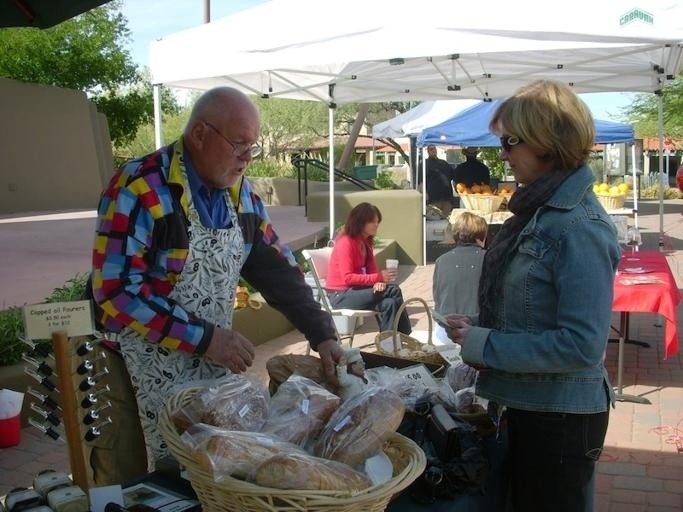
[462,147,480,155]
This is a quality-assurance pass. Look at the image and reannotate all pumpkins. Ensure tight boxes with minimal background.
[457,183,515,195]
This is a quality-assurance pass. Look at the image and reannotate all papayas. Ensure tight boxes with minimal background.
[593,183,629,195]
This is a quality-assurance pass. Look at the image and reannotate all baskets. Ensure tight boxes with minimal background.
[460,193,504,212]
[596,193,626,209]
[159,297,487,512]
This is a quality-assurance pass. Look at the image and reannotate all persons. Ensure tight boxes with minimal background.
[85,86,346,475]
[453,146,489,188]
[432,211,487,342]
[443,79,621,511]
[412,144,453,204]
[324,202,412,336]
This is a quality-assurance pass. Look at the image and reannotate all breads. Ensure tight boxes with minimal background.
[173,375,405,490]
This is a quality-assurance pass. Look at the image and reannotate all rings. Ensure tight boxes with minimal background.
[229,364,239,371]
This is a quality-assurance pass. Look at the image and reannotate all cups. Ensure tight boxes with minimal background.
[386,259,399,281]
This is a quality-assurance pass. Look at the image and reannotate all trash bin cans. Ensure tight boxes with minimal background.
[353,165,378,180]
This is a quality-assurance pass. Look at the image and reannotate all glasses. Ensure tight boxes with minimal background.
[500,137,523,150]
[205,122,262,158]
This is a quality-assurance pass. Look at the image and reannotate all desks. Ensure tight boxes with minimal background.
[601,246,673,406]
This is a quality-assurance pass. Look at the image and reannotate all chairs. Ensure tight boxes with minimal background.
[301,244,385,344]
